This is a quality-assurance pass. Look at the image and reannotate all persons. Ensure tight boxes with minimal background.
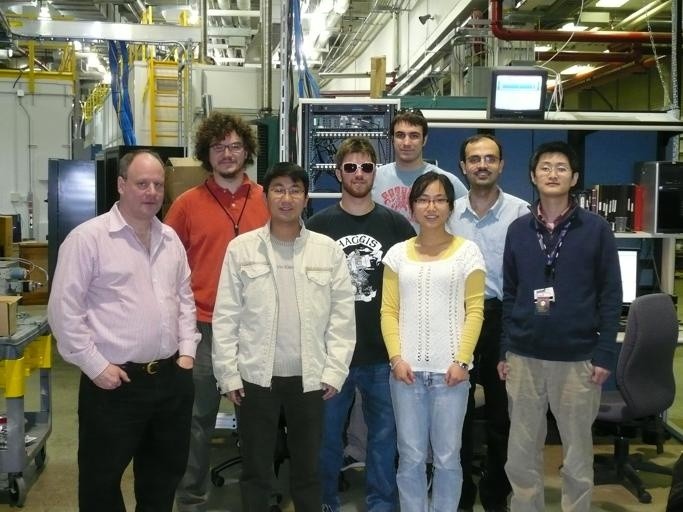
[163,110,271,512]
[212,163,357,512]
[46,150,202,512]
[498,143,623,512]
[444,134,532,512]
[380,171,487,512]
[304,139,417,511]
[339,114,469,493]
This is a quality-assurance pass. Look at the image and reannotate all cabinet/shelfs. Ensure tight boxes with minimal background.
[0,214,48,304]
[421,110,683,131]
[613,232,683,444]
[289,98,401,219]
[1,305,53,508]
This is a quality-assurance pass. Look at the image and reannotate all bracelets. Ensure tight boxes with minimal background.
[389,355,403,371]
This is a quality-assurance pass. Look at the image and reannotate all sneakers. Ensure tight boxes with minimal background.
[340,453,366,471]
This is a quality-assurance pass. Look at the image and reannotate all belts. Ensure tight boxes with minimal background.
[123,358,170,374]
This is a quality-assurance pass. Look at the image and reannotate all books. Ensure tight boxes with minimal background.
[572,183,646,232]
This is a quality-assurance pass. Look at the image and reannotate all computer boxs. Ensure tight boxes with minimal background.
[638,159,683,234]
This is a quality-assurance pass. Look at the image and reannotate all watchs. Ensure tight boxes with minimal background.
[452,360,469,373]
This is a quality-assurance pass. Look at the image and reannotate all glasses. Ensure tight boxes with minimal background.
[398,107,423,115]
[467,156,500,164]
[340,162,376,174]
[541,166,573,173]
[208,143,244,153]
[269,186,305,198]
[414,198,451,206]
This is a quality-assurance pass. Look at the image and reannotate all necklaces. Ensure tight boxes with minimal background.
[204,178,251,237]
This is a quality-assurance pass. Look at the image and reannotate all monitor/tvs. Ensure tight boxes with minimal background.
[615,246,642,316]
[488,69,548,123]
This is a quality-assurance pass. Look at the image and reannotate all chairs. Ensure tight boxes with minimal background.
[594,294,679,503]
